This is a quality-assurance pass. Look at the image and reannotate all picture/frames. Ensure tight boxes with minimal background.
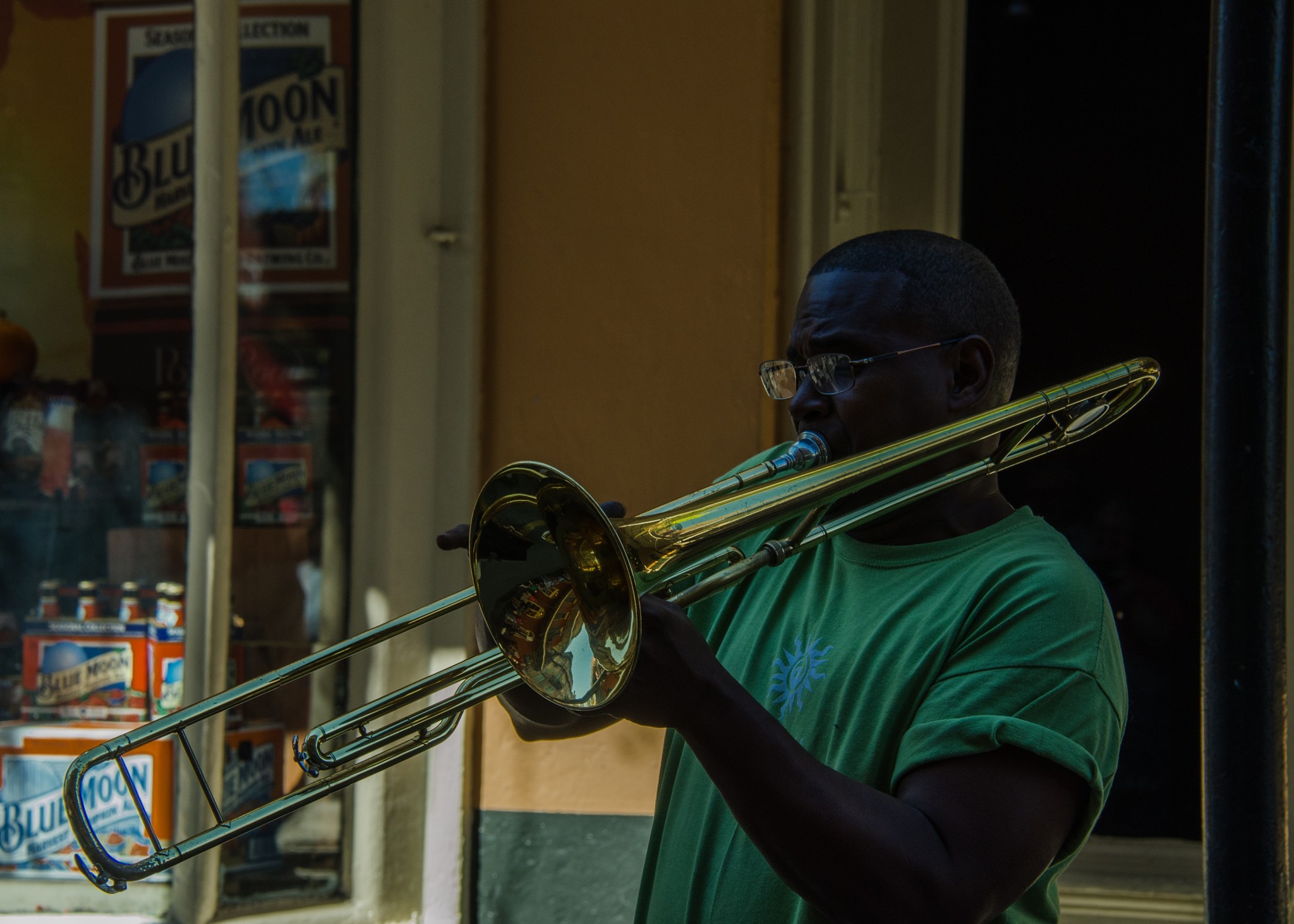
[81,0,358,313]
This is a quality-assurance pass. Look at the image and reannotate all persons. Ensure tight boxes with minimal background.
[437,229,1127,924]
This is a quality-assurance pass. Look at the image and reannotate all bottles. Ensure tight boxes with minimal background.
[119,581,145,621]
[38,579,61,616]
[155,581,187,628]
[75,580,98,619]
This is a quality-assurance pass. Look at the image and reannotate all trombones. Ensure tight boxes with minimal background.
[58,348,1168,896]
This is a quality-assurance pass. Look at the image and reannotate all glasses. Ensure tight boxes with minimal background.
[758,334,982,400]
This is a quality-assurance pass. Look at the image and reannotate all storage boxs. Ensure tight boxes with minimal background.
[219,718,288,825]
[135,419,198,531]
[137,620,196,725]
[0,718,178,881]
[231,425,323,538]
[11,612,149,725]
